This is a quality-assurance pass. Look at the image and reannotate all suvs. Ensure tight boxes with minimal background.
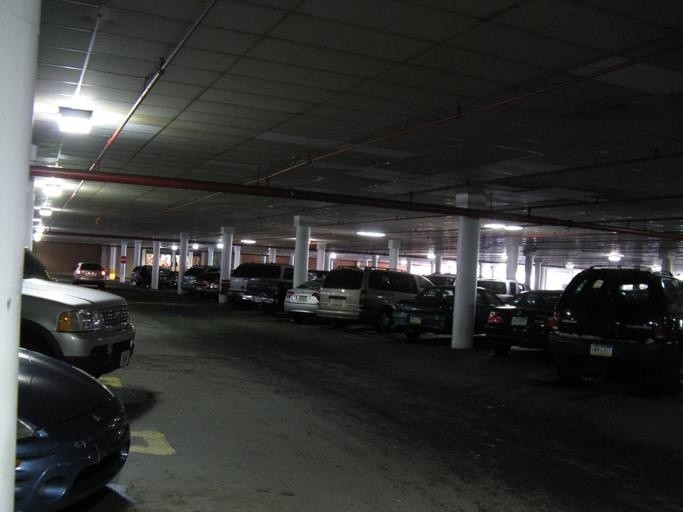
[543,266,683,394]
[315,265,438,333]
[130,261,331,309]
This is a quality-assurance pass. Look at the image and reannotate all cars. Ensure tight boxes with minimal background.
[429,272,530,303]
[283,279,322,319]
[392,286,507,339]
[487,291,563,350]
[14,246,136,512]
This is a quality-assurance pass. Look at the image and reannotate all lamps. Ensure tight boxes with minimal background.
[42,184,63,198]
[38,200,52,218]
[608,255,622,262]
[56,98,93,135]
